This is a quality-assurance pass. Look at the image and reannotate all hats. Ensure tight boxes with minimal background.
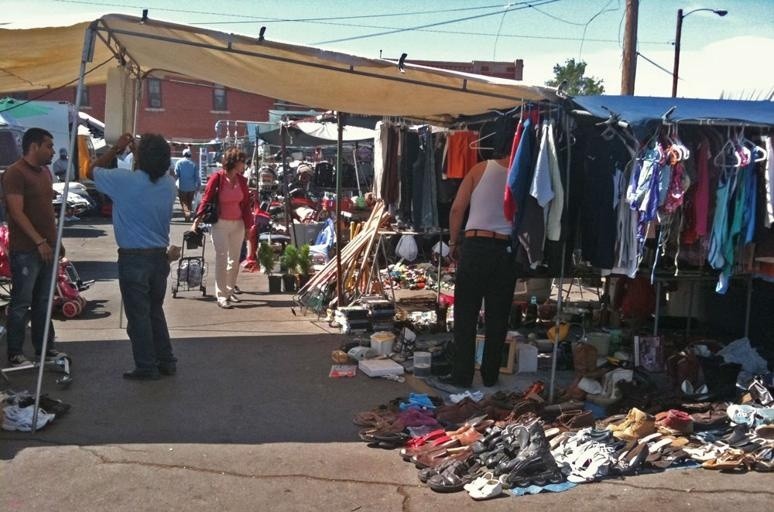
[59,148,67,156]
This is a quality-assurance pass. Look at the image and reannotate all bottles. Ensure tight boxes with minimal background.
[323,190,364,209]
[599,302,607,321]
[529,295,537,316]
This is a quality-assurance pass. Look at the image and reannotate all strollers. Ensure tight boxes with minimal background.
[0,223,14,319]
[51,253,96,319]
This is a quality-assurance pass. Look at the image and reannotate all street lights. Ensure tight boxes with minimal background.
[670,7,728,98]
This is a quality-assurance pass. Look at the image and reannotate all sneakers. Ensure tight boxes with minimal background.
[156,359,178,377]
[32,348,68,361]
[121,364,159,382]
[69,214,81,222]
[216,299,234,309]
[227,294,240,303]
[181,148,192,156]
[232,285,241,294]
[64,216,71,222]
[7,351,34,368]
[0,388,71,434]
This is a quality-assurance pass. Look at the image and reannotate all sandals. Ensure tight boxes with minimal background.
[349,347,774,502]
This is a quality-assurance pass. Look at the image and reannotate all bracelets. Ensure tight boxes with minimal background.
[111,145,123,156]
[447,238,458,247]
[34,238,48,246]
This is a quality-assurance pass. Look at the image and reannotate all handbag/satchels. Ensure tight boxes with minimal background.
[193,171,221,225]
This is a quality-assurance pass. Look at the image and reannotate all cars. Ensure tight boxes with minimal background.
[50,182,90,219]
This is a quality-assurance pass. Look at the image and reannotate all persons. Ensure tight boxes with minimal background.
[438,152,528,388]
[53,148,75,182]
[174,147,201,222]
[86,133,178,379]
[1,128,73,367]
[195,147,255,309]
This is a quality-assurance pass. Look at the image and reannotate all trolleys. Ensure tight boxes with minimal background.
[170,226,210,299]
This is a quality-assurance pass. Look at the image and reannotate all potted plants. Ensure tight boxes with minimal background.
[298,242,312,291]
[254,238,282,295]
[279,243,297,292]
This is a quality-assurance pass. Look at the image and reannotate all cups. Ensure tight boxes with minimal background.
[412,351,431,378]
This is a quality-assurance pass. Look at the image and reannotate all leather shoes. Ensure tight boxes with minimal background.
[463,227,510,243]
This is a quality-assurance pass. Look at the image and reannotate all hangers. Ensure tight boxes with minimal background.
[467,98,773,172]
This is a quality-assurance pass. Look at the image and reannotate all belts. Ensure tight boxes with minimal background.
[117,247,167,255]
